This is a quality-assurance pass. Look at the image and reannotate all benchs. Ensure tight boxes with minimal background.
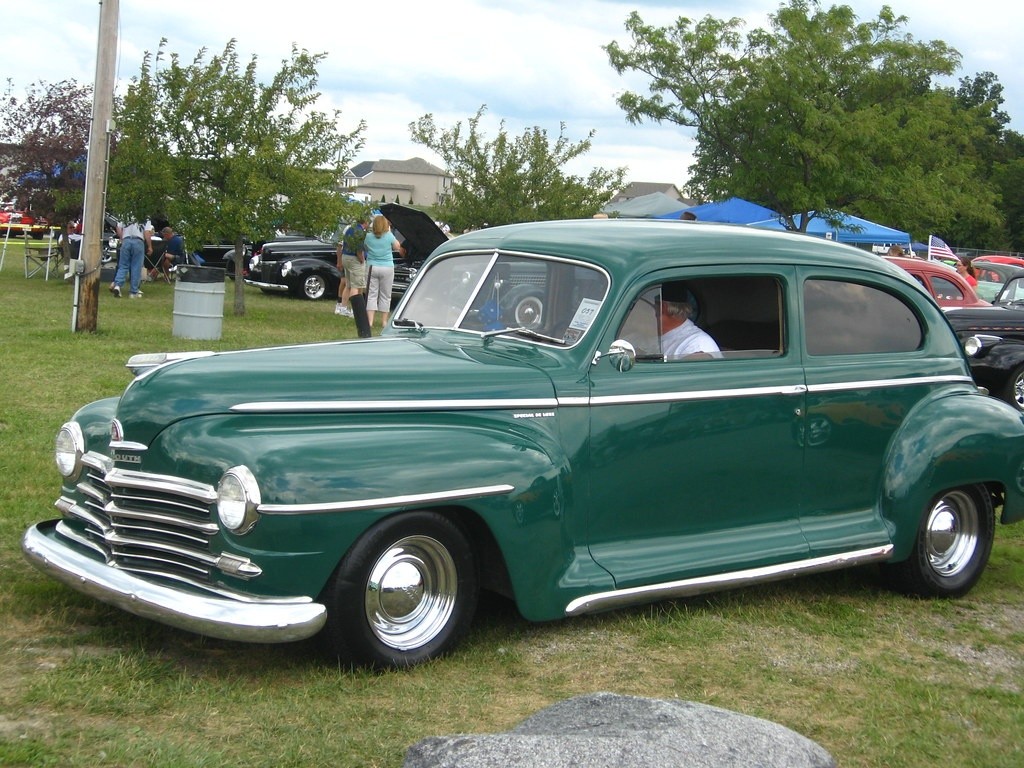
[704,321,780,351]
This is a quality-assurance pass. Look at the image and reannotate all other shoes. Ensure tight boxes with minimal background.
[113,285,122,299]
[129,293,142,300]
[340,308,354,318]
[138,290,144,295]
[108,281,115,292]
[334,303,341,314]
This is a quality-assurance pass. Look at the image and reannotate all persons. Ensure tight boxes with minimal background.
[887,245,904,256]
[58,211,187,298]
[956,257,978,295]
[337,215,401,327]
[680,210,697,220]
[619,285,724,361]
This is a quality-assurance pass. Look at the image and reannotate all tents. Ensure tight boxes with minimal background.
[0,152,88,280]
[603,191,928,258]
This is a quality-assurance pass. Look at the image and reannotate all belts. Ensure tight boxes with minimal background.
[123,236,144,241]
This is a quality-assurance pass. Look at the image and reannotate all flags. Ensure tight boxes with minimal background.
[929,235,961,262]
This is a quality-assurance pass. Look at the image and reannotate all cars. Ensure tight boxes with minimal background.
[0,201,51,240]
[20,214,1024,666]
[883,253,1024,410]
[241,215,352,301]
[389,253,428,301]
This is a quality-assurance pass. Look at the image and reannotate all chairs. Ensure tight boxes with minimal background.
[140,240,171,284]
[52,231,81,270]
[24,247,60,279]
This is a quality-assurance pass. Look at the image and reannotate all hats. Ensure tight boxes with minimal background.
[659,280,695,304]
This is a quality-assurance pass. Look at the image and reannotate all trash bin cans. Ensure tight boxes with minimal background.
[172,264,227,341]
